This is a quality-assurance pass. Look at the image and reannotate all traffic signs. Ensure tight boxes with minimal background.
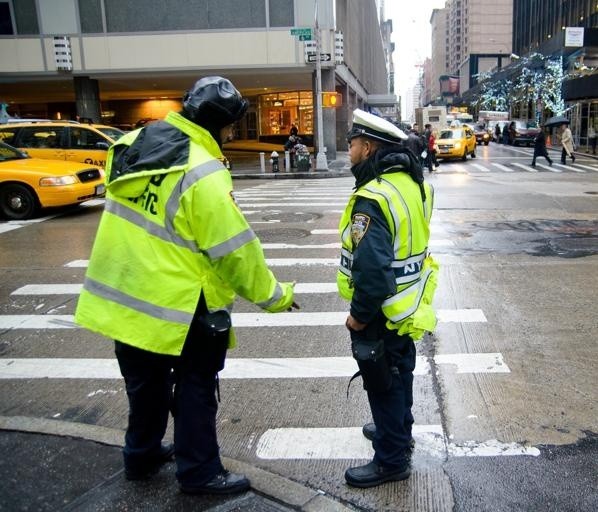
[290,28,312,36]
[299,35,312,40]
[308,54,330,61]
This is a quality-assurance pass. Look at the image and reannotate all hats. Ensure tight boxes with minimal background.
[345,107,409,146]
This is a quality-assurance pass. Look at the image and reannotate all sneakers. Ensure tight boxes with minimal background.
[526,158,576,167]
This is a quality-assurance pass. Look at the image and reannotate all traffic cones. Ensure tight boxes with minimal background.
[545,135,551,148]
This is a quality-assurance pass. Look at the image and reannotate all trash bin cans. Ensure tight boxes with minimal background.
[295,154,310,171]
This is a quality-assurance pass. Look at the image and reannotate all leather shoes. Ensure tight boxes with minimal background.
[121,440,177,480]
[344,456,413,489]
[179,470,252,498]
[362,422,382,442]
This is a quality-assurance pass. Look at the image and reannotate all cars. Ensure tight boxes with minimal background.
[0,119,128,220]
[415,106,541,161]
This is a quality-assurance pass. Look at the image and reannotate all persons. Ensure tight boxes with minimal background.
[336,106,440,490]
[502,122,509,146]
[528,126,554,169]
[283,123,311,173]
[393,122,440,174]
[559,123,577,165]
[495,123,501,143]
[72,73,301,496]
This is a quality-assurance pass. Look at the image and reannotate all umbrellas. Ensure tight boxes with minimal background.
[543,116,571,128]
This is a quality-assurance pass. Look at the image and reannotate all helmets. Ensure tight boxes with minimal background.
[182,75,249,130]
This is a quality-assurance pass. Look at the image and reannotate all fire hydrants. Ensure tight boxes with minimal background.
[269,151,280,173]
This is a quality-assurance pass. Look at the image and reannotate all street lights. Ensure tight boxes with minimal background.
[505,52,564,146]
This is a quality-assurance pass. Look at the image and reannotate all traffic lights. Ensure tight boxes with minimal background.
[323,92,343,107]
